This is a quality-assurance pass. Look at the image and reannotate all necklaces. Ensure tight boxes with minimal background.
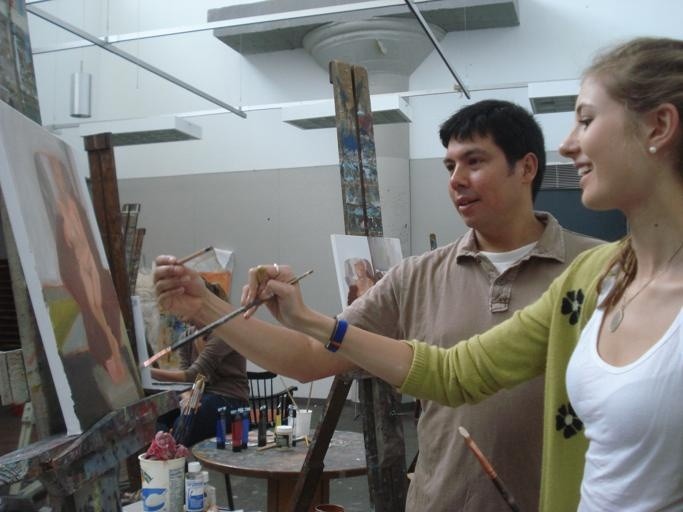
[609,244,682,333]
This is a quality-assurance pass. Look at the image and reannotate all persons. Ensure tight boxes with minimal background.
[240,37,682,511]
[351,261,374,299]
[46,158,124,386]
[149,276,249,449]
[150,98,610,511]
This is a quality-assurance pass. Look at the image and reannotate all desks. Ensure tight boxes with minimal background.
[192,428,368,511]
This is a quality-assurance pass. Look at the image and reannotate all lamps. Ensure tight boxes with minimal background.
[69,59,92,119]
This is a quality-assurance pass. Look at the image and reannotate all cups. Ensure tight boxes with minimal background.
[295,409,313,439]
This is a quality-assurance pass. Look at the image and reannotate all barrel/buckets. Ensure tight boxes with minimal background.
[288,409,312,437]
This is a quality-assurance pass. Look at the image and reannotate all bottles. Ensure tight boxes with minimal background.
[185,461,205,512]
[201,471,216,512]
[275,426,293,450]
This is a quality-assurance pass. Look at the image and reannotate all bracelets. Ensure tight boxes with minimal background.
[324,320,349,353]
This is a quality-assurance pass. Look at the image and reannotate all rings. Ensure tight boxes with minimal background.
[272,263,280,280]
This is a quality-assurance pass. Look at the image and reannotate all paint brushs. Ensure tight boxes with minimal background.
[139,269,314,371]
[307,380,313,412]
[176,246,213,265]
[281,378,298,411]
[152,383,194,385]
[173,374,206,443]
[459,425,520,512]
[258,435,305,451]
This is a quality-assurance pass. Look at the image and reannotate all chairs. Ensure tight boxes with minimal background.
[243,371,296,427]
